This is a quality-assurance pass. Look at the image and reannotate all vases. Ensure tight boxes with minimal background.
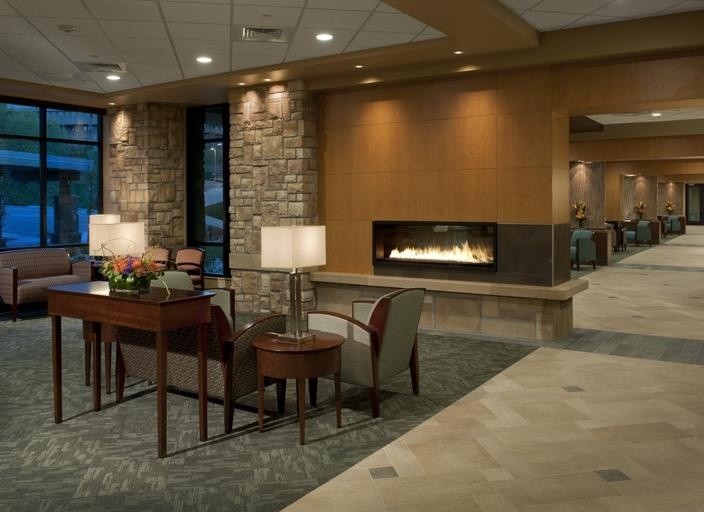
[639,212,644,219]
[579,219,583,228]
[110,276,150,295]
[669,210,673,216]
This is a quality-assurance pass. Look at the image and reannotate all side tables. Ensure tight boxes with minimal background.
[253,328,348,443]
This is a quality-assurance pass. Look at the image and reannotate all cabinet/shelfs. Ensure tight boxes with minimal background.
[626,218,662,244]
[573,224,612,264]
[657,213,686,232]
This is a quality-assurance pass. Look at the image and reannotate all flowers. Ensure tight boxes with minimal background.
[89,237,171,291]
[635,201,649,215]
[666,200,674,212]
[571,201,586,220]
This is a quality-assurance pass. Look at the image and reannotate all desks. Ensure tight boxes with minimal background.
[43,281,217,459]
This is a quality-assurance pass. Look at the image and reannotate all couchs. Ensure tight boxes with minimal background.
[173,248,206,289]
[116,271,286,417]
[571,228,598,272]
[144,244,171,274]
[626,219,652,246]
[0,249,93,323]
[307,286,425,419]
[665,213,681,236]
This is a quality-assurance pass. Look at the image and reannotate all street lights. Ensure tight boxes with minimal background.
[210,143,221,181]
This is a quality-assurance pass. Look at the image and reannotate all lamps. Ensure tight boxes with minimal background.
[89,212,120,227]
[88,223,147,259]
[261,226,329,345]
